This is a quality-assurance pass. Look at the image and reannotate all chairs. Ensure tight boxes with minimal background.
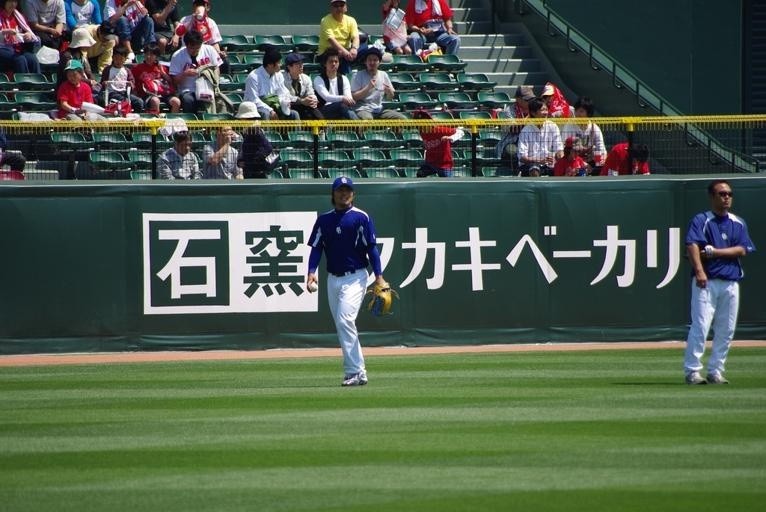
[0,34,552,179]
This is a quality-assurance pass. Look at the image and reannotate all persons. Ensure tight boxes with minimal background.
[0,0,228,119]
[493,80,652,178]
[0,130,26,179]
[154,102,281,178]
[243,48,408,130]
[319,1,462,59]
[414,108,468,177]
[683,178,756,386]
[306,176,387,387]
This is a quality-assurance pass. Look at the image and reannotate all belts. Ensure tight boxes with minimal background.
[330,270,355,276]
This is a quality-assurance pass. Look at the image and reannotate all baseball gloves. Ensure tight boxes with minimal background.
[367,281,392,317]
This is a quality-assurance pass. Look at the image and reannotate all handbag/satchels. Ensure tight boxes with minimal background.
[143,77,176,96]
[105,99,131,116]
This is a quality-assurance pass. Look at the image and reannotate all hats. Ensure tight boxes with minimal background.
[100,20,117,40]
[333,177,353,190]
[235,102,260,119]
[286,53,305,64]
[517,86,536,100]
[541,84,555,97]
[566,136,587,151]
[69,27,96,49]
[63,59,84,72]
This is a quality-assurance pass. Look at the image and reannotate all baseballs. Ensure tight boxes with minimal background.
[310,282,319,294]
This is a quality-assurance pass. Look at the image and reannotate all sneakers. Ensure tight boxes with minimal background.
[342,371,367,385]
[686,372,707,384]
[123,53,137,64]
[709,373,729,384]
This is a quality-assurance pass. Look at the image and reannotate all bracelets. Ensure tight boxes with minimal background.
[704,244,716,259]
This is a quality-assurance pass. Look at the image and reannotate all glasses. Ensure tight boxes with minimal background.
[713,190,733,198]
